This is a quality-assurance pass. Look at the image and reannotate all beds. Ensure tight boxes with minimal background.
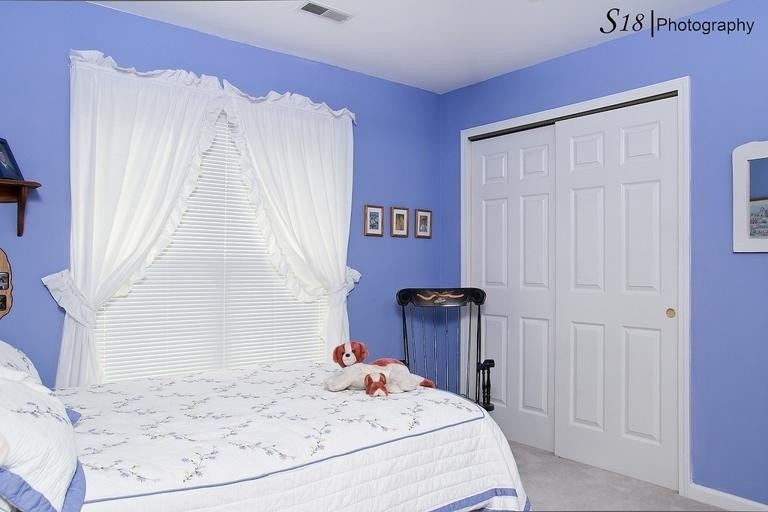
[0,359,531,512]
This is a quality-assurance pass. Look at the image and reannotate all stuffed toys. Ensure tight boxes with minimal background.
[322,341,437,398]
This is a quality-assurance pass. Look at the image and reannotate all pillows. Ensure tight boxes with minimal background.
[0,341,43,384]
[0,364,86,512]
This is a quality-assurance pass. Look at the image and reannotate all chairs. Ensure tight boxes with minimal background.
[396,287,494,411]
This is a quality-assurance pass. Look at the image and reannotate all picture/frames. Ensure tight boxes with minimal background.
[364,204,384,237]
[732,140,768,253]
[390,206,409,238]
[415,209,432,239]
[0,137,25,180]
